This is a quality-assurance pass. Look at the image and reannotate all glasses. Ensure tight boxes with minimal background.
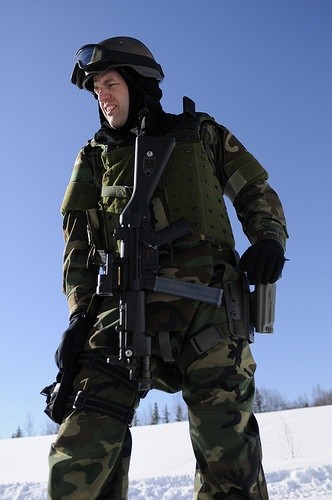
[70,44,164,85]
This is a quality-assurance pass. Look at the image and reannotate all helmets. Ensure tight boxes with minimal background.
[81,37,164,100]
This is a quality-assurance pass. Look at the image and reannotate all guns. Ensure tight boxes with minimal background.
[94,108,225,392]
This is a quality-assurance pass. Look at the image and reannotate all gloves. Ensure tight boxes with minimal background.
[53,312,93,376]
[237,238,285,285]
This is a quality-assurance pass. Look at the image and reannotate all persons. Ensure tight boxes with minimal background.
[41,37,290,500]
